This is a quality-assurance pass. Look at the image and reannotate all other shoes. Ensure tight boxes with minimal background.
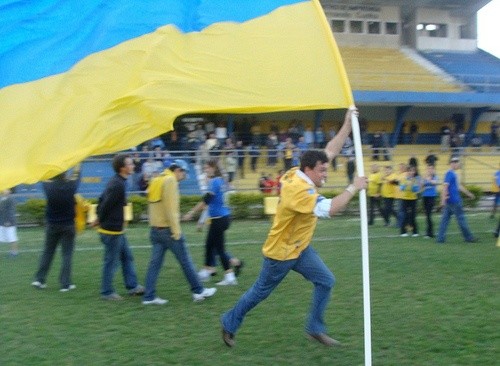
[401,234,408,237]
[192,287,217,302]
[142,296,168,305]
[210,271,217,276]
[305,329,343,347]
[59,283,76,292]
[215,273,237,287]
[101,292,123,301]
[31,279,47,288]
[411,234,419,237]
[221,328,235,347]
[197,271,211,282]
[467,237,482,243]
[423,236,429,239]
[234,258,244,277]
[127,285,145,294]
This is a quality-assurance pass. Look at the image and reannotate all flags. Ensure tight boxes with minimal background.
[0,0,357,188]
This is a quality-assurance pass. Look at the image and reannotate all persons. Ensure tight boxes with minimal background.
[366,154,441,240]
[128,117,423,193]
[140,158,220,306]
[436,157,481,244]
[217,104,368,350]
[183,158,246,286]
[0,187,20,260]
[92,153,148,302]
[491,216,500,239]
[439,118,500,163]
[489,162,500,220]
[29,161,82,293]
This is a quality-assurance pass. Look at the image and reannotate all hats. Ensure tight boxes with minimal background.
[451,157,459,163]
[171,160,192,172]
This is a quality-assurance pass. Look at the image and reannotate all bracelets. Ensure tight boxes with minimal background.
[188,209,196,219]
[344,184,358,197]
